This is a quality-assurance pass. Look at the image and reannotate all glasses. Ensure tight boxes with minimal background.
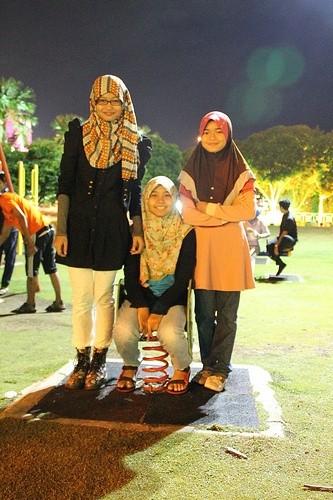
[96,99,120,106]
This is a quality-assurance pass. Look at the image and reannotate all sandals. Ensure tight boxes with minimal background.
[116,365,139,392]
[166,366,190,394]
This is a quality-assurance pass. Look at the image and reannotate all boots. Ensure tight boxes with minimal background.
[84,346,107,389]
[64,346,91,389]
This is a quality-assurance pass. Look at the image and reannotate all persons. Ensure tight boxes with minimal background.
[53,75,152,392]
[266,198,298,277]
[1,192,66,314]
[241,207,270,277]
[0,173,19,296]
[177,111,256,391]
[114,174,196,396]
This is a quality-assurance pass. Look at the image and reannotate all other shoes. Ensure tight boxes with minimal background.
[191,369,211,384]
[276,262,286,276]
[204,373,226,391]
[44,300,65,312]
[14,303,36,313]
